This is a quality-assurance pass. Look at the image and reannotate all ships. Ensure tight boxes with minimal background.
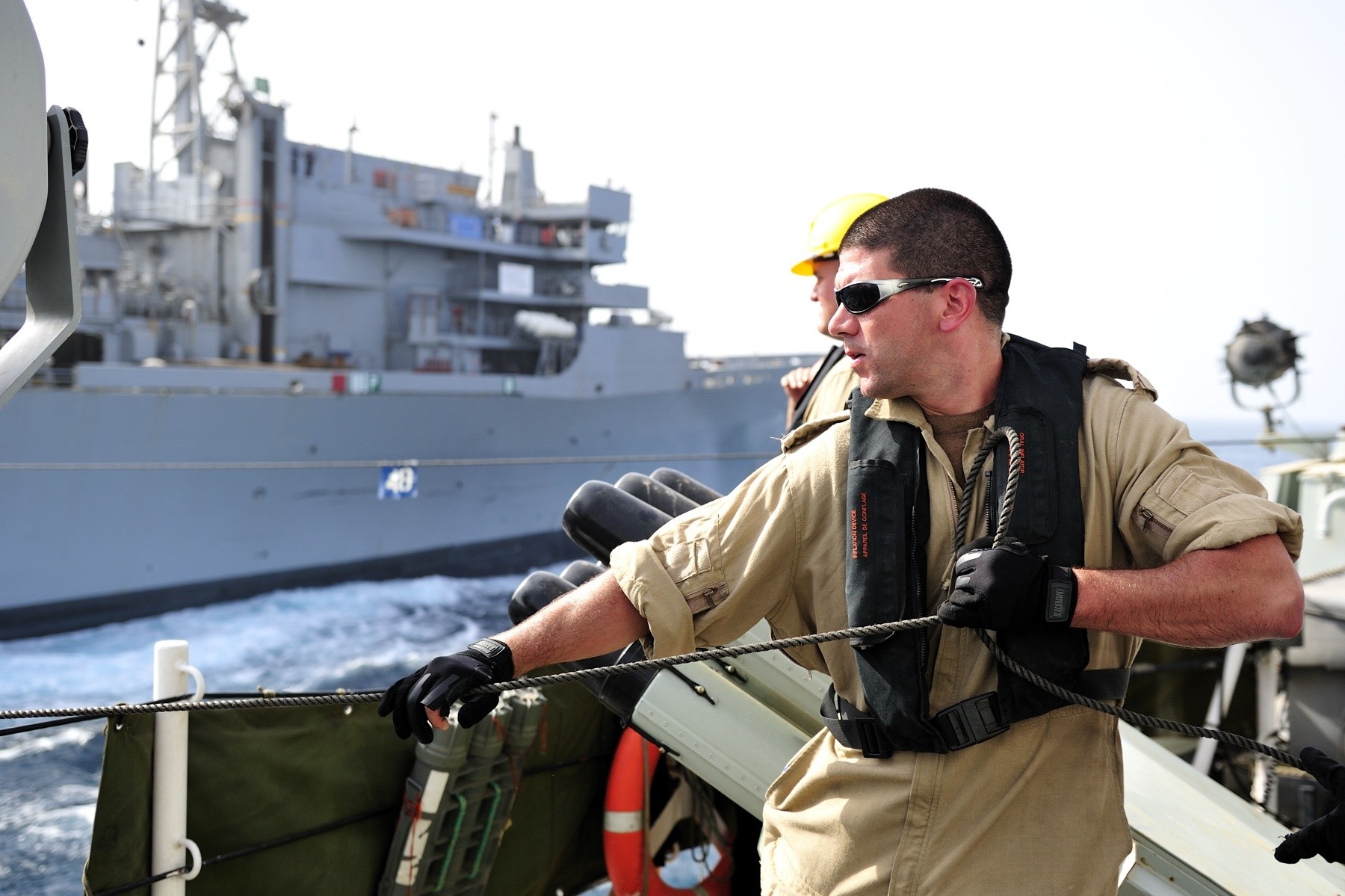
[0,0,832,643]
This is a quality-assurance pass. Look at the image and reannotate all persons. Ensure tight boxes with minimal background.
[378,188,1305,895]
[779,191,890,436]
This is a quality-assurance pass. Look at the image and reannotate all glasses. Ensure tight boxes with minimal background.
[833,275,986,315]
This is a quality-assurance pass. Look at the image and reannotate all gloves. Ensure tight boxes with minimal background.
[1273,745,1345,865]
[936,535,1079,633]
[376,636,516,745]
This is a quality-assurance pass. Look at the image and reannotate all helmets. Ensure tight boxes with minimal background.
[790,191,891,277]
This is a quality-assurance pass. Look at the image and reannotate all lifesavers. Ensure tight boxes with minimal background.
[602,724,736,895]
